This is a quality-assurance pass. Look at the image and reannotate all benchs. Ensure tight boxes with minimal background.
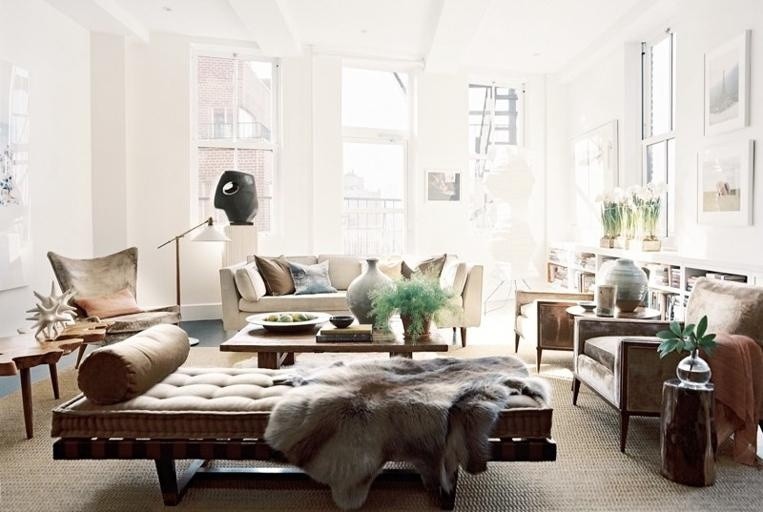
[51,368,556,512]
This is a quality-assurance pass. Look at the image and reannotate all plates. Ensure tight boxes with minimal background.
[246,312,330,334]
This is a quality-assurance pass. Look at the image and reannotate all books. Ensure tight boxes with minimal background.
[317,322,373,342]
[548,252,746,322]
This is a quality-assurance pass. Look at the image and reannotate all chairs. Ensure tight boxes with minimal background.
[47,247,180,369]
[514,289,647,374]
[572,276,763,463]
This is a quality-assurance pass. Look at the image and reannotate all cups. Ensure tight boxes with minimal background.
[595,285,615,319]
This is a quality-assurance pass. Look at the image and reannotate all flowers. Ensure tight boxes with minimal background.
[595,183,671,241]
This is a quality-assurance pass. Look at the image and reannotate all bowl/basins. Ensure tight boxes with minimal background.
[581,304,596,312]
[329,316,354,330]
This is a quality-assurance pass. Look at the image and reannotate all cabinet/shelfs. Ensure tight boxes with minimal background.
[547,242,748,322]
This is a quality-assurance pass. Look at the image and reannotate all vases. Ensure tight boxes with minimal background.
[598,238,662,252]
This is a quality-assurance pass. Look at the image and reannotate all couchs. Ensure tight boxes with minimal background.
[219,262,483,346]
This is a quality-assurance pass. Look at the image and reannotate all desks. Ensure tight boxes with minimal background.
[565,305,661,391]
[0,322,116,439]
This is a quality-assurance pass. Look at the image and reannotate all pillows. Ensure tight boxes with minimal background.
[361,256,401,289]
[401,254,447,281]
[318,254,360,290]
[73,288,145,320]
[439,262,467,296]
[287,260,337,294]
[77,323,190,406]
[246,255,315,266]
[235,261,266,302]
[254,255,295,296]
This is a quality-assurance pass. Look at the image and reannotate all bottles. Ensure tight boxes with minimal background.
[600,258,651,317]
[346,258,397,327]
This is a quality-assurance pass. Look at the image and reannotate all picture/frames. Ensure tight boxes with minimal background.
[425,170,463,206]
[697,139,755,225]
[703,30,751,137]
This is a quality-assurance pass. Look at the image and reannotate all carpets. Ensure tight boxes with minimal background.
[0,345,763,512]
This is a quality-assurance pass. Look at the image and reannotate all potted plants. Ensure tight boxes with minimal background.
[656,315,718,386]
[367,261,462,346]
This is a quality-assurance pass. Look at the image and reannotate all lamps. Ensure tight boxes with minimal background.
[156,216,232,346]
